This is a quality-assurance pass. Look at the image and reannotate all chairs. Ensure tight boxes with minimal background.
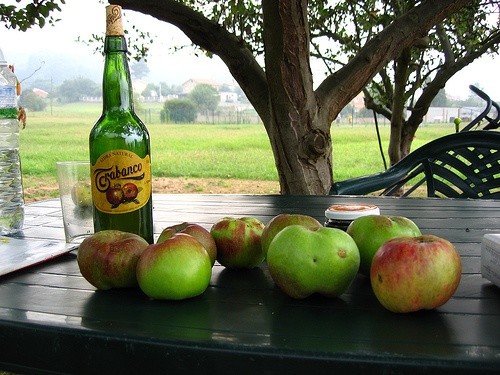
[328,130,500,199]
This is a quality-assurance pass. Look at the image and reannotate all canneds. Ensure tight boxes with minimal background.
[324,205,380,231]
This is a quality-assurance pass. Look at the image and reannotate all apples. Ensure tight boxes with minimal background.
[346,214,422,274]
[76,229,150,290]
[267,224,361,299]
[259,214,325,260]
[106,183,139,205]
[156,222,217,266]
[209,217,267,269]
[136,233,211,300]
[370,234,461,313]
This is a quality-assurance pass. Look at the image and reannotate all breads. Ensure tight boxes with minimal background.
[71,180,93,207]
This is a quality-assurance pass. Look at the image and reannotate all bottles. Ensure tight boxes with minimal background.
[1,49,24,237]
[323,203,380,231]
[89,4,154,246]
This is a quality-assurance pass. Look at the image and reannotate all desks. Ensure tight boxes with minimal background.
[0,192,500,375]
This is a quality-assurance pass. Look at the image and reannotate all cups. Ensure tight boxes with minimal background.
[56,160,95,255]
